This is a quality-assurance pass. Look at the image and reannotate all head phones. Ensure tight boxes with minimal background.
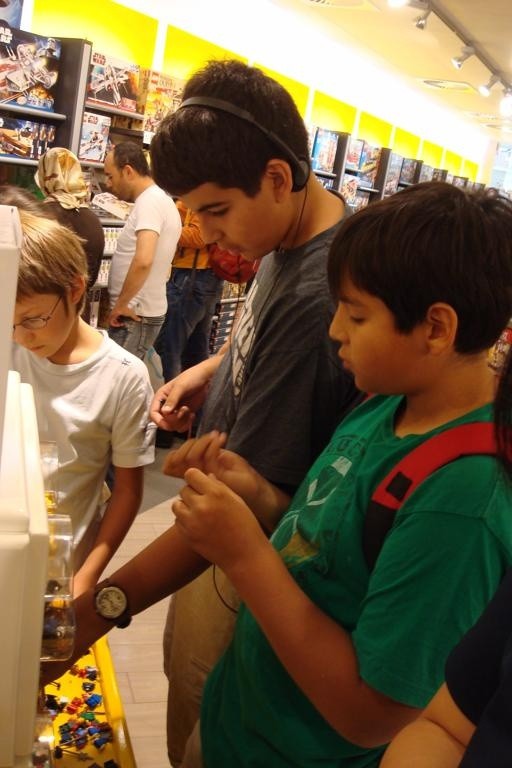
[178,88,313,199]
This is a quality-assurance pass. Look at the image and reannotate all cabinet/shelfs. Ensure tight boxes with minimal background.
[307,125,487,214]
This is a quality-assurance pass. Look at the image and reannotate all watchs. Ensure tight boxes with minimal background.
[94,578,132,629]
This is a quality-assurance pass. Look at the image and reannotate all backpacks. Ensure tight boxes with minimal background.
[207,243,259,284]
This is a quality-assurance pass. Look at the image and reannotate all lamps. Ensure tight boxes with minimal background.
[411,0,511,98]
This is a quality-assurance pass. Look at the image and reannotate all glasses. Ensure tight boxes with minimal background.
[13,295,62,334]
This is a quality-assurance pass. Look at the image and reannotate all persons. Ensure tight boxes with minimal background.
[9,209,155,601]
[104,142,183,361]
[152,198,225,449]
[34,147,105,325]
[379,345,512,767]
[39,59,357,768]
[162,182,512,767]
